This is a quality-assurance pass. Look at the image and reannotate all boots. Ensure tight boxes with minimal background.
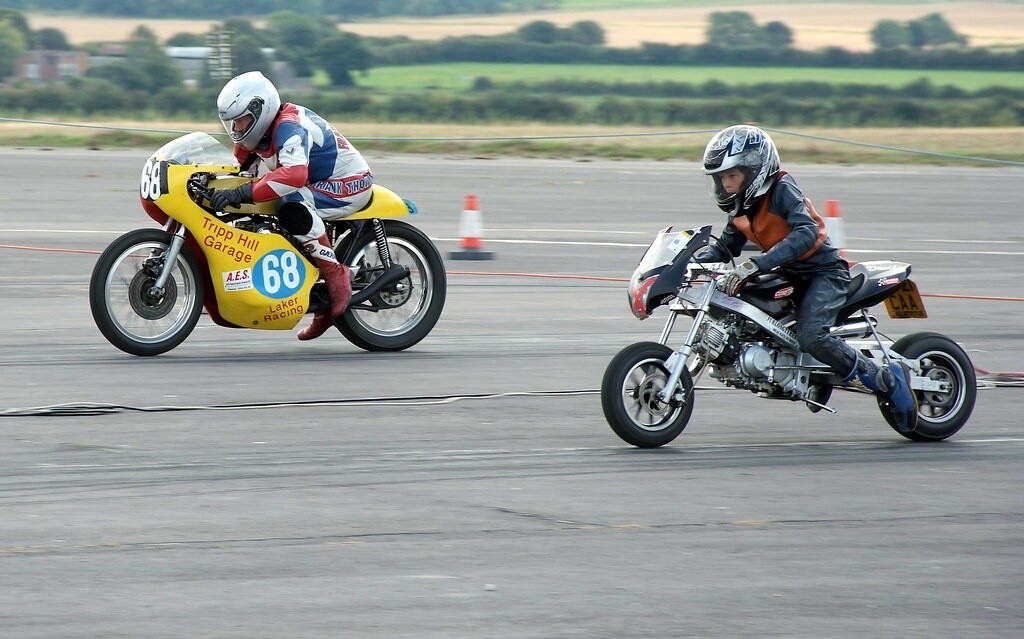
[302,233,355,317]
[835,348,918,432]
[297,314,333,341]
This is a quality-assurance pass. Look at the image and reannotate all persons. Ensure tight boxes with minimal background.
[210,71,373,341]
[690,125,919,433]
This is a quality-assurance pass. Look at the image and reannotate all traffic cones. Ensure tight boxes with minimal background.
[820,196,859,268]
[443,193,496,260]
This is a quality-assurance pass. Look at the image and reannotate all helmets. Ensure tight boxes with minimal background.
[216,71,281,153]
[702,125,780,217]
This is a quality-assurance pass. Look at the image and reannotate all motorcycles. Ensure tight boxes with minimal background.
[89,131,448,356]
[601,223,977,448]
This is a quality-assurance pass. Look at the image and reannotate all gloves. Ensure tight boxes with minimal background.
[720,259,761,298]
[208,180,257,213]
